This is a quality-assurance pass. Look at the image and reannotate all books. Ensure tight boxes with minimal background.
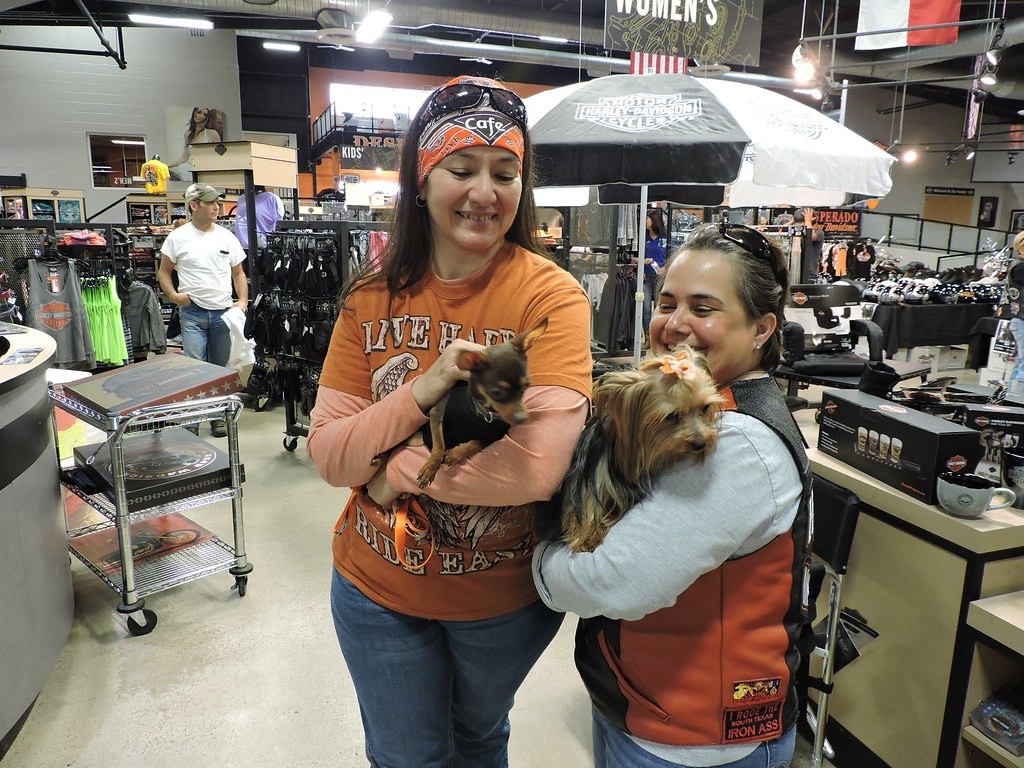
[969,679,1024,756]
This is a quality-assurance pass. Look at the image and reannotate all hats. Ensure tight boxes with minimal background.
[185,183,226,202]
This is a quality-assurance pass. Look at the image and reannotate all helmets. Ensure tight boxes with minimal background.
[794,207,816,224]
[817,269,1002,304]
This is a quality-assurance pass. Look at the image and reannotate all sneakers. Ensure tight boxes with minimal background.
[211,420,227,437]
[183,423,198,436]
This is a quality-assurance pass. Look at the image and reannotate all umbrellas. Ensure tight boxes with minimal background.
[522,74,898,367]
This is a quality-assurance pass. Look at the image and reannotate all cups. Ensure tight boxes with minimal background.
[880,434,890,460]
[890,438,902,464]
[858,426,867,452]
[869,430,879,456]
[936,471,1017,521]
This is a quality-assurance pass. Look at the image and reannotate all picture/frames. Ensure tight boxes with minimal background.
[1009,209,1024,233]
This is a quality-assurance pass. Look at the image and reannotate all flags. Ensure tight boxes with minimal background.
[630,51,687,75]
[854,0,962,50]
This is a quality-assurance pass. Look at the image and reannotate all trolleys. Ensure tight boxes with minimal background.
[48,380,254,636]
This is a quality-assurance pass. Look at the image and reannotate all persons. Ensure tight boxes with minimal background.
[1007,232,1024,394]
[307,78,592,768]
[236,186,285,250]
[531,231,815,768]
[168,107,223,168]
[634,211,677,350]
[159,183,249,437]
[141,155,170,195]
[783,208,824,284]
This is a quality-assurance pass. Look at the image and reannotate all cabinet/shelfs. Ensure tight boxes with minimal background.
[956,588,1024,768]
[55,223,193,323]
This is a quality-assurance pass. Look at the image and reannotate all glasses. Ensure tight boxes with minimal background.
[706,222,780,283]
[415,84,527,139]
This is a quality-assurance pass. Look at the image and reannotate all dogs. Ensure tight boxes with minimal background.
[535,343,731,554]
[402,317,549,491]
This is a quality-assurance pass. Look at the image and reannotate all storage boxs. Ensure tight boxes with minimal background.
[191,144,298,188]
[817,380,1024,505]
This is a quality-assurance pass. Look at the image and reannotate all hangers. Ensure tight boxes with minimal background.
[11,233,133,285]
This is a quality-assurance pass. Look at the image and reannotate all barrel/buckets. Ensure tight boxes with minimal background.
[1000,448,1024,512]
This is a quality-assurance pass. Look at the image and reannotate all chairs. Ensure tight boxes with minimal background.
[798,471,861,768]
[780,284,886,406]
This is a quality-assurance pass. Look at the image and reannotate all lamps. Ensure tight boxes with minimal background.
[973,42,1003,104]
[128,11,213,30]
